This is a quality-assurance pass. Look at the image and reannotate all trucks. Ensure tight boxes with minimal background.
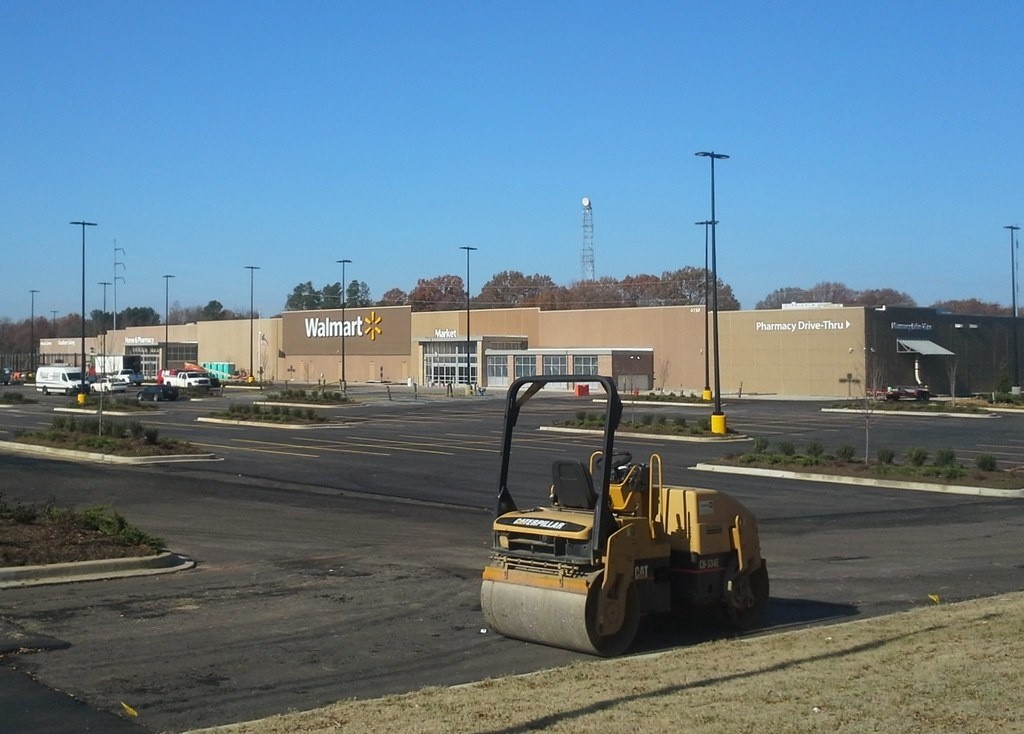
[157,368,221,389]
[84,354,142,385]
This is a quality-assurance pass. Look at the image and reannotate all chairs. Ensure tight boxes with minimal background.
[553,459,613,510]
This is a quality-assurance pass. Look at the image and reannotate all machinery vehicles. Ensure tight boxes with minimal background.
[480,373,770,661]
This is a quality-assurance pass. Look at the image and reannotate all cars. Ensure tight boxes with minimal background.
[89,377,129,394]
[135,385,179,402]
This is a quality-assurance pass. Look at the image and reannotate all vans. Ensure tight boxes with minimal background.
[35,363,91,397]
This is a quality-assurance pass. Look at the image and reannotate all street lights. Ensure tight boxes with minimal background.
[98,282,113,377]
[162,274,175,369]
[28,290,41,381]
[335,260,352,392]
[694,220,720,401]
[69,221,98,406]
[243,266,261,383]
[50,310,59,338]
[458,246,479,397]
[1003,225,1022,388]
[694,151,730,434]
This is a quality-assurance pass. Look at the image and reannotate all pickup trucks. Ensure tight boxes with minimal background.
[163,371,211,392]
[106,368,144,386]
